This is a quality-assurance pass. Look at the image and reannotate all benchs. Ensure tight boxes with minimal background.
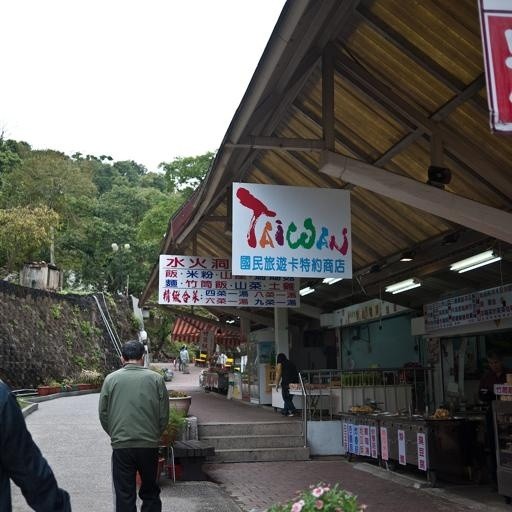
[171,440,215,482]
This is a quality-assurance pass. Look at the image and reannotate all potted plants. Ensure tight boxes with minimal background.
[157,403,189,445]
[35,368,105,396]
[165,390,192,415]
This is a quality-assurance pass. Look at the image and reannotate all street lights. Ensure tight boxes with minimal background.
[111,243,131,292]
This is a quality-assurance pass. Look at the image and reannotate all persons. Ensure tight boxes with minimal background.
[476,346,512,494]
[0,378,74,512]
[274,352,299,417]
[99,340,170,512]
[180,346,190,370]
[174,344,188,368]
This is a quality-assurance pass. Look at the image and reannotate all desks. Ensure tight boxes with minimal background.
[268,383,332,420]
[339,411,489,483]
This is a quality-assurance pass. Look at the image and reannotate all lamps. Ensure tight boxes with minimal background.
[383,249,502,295]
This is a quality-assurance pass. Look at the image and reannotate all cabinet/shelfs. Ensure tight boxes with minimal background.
[491,400,512,505]
